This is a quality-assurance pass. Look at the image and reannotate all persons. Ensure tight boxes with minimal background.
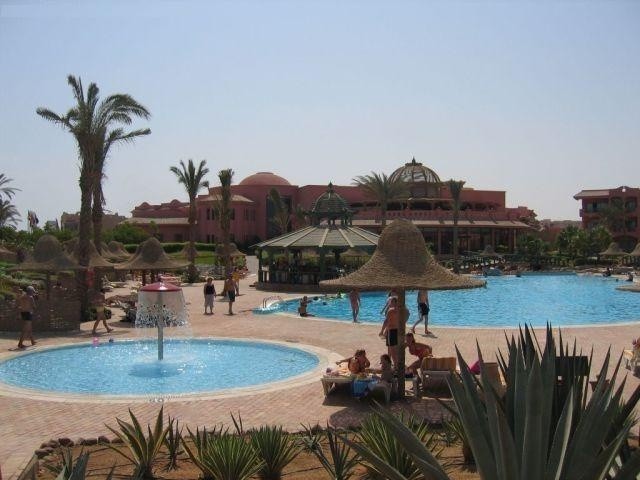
[301,296,308,308]
[370,353,429,381]
[378,295,400,372]
[297,302,313,316]
[625,272,633,282]
[224,273,240,316]
[379,293,394,317]
[602,267,611,278]
[90,287,114,335]
[49,281,67,310]
[202,278,216,316]
[336,349,371,375]
[349,286,361,322]
[15,285,40,349]
[402,332,434,376]
[410,289,432,335]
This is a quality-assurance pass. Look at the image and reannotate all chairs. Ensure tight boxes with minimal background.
[622,336,640,376]
[321,357,456,406]
